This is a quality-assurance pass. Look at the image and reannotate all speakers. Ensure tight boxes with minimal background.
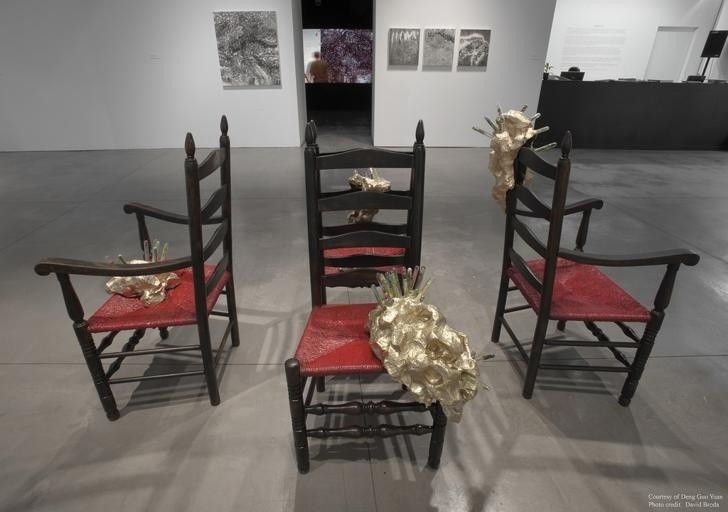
[701,31,728,57]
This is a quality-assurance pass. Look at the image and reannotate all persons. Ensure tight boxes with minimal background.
[306,52,329,83]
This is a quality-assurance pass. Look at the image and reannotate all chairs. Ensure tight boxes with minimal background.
[32,113,700,475]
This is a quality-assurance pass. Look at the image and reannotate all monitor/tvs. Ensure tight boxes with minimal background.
[688,76,706,83]
[561,72,584,81]
